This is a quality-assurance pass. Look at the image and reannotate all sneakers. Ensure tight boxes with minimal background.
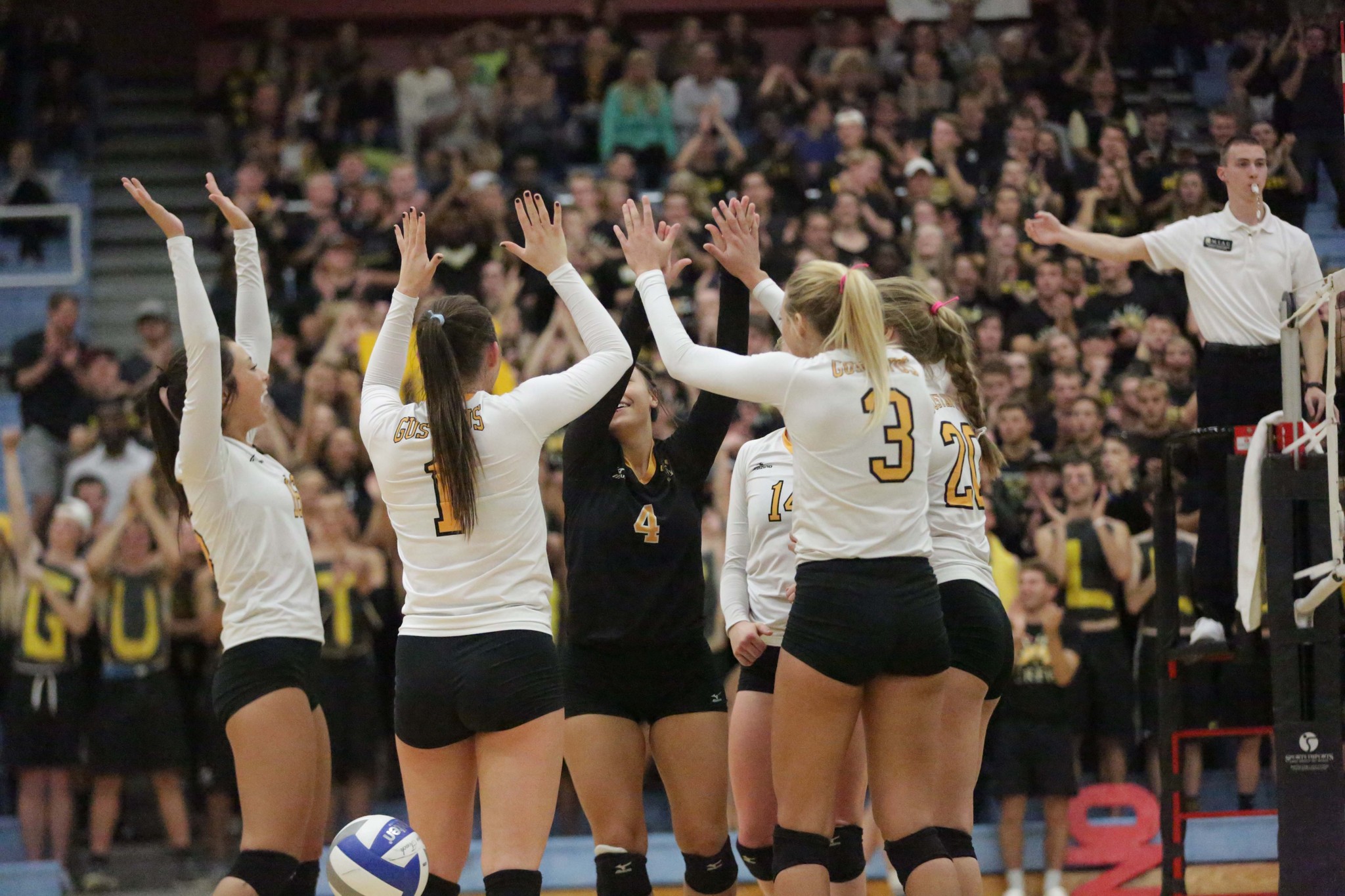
[1190,614,1226,649]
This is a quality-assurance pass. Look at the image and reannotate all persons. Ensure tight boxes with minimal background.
[0,0,1345,896]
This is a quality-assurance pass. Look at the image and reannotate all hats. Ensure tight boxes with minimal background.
[55,497,95,528]
[903,156,936,178]
[1025,449,1061,469]
[463,169,501,192]
[833,110,865,125]
[136,298,170,319]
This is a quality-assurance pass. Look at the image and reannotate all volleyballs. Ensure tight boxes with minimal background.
[326,814,429,896]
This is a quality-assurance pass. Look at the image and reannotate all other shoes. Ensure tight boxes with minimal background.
[1002,882,1067,896]
[167,853,209,892]
[80,855,118,893]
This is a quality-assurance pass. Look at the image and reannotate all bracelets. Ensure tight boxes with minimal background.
[1305,382,1325,390]
[1092,517,1105,528]
